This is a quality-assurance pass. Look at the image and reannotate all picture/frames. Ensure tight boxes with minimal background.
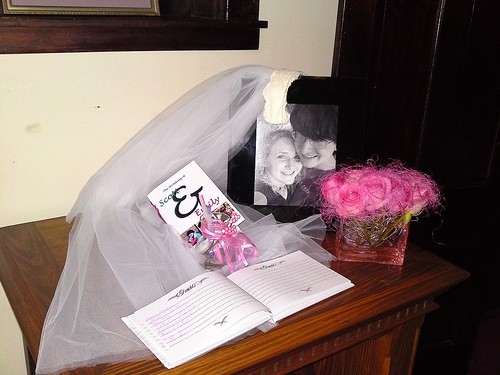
[228,76,370,230]
[2,0,160,16]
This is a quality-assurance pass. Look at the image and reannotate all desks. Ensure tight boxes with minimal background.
[0,216,471,375]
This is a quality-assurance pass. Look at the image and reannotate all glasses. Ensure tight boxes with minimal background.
[292,131,333,150]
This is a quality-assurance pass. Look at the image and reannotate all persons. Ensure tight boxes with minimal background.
[254,104,338,205]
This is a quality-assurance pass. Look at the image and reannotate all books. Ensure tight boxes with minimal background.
[120,160,355,370]
